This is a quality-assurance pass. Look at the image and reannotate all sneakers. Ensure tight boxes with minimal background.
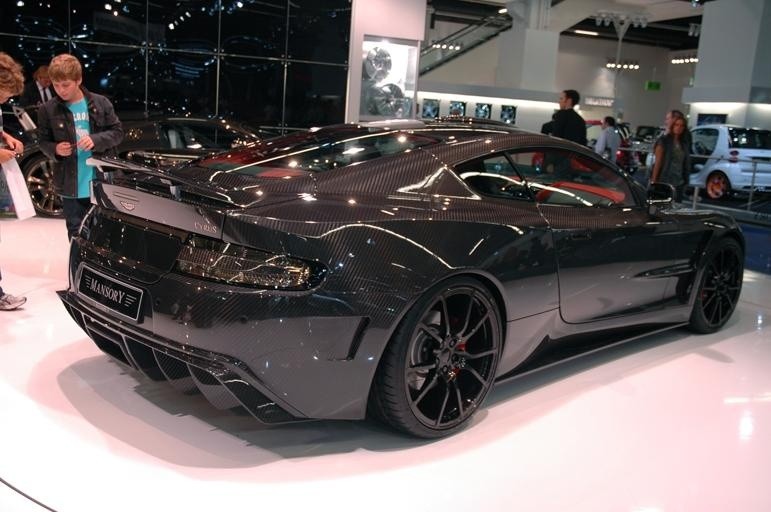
[0,293,26,310]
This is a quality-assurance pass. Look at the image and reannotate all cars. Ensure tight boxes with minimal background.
[645,123,771,199]
[533,120,639,176]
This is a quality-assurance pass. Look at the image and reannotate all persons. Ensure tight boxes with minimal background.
[25,53,123,247]
[593,112,630,172]
[0,51,27,310]
[644,107,700,204]
[540,84,596,180]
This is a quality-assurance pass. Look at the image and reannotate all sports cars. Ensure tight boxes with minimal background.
[16,117,263,218]
[57,115,745,441]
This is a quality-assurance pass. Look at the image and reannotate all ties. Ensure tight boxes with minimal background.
[43,87,48,102]
